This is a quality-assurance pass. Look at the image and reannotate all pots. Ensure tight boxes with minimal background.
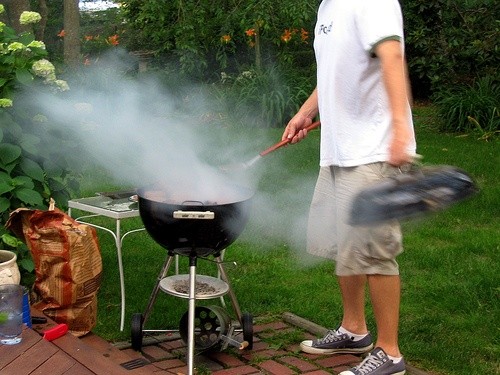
[138,184,254,256]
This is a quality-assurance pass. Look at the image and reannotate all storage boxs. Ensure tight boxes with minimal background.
[4,210,103,338]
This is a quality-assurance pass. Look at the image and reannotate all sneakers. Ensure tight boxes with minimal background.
[299,331,374,354]
[338,347,407,375]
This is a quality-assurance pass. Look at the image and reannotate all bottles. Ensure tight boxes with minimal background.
[23,285,32,328]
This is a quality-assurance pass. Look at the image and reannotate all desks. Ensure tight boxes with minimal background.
[68,196,146,333]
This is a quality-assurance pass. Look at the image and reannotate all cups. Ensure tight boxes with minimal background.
[0,284,23,345]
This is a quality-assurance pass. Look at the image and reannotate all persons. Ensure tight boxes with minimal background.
[280,0,422,375]
